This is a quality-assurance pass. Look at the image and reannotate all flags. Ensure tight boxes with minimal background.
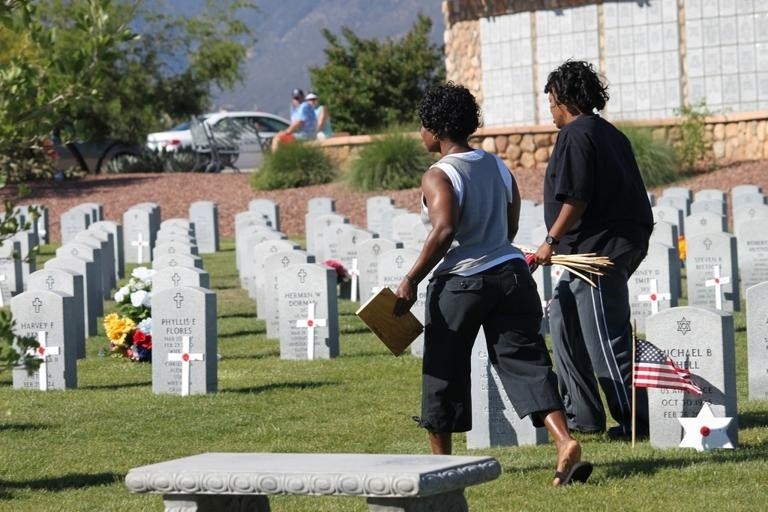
[634,336,704,396]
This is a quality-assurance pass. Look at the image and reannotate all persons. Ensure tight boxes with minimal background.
[305,91,333,140]
[534,53,654,440]
[270,88,317,156]
[393,78,581,486]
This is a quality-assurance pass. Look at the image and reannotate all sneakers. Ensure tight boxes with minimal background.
[565,416,605,436]
[609,426,649,441]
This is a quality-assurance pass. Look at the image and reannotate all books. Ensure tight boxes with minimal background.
[355,286,425,359]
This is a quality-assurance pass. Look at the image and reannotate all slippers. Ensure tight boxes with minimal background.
[547,462,594,487]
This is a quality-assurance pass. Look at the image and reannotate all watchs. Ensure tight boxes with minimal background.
[546,235,561,247]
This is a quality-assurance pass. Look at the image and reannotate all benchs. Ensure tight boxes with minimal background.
[189,120,240,173]
[125,451,500,512]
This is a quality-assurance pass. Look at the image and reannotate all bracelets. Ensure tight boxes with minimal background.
[403,274,421,287]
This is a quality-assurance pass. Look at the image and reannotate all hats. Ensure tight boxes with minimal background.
[291,88,319,101]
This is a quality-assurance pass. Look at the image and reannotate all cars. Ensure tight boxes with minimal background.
[46,135,160,173]
[146,111,305,172]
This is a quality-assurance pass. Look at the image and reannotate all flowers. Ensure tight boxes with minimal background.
[321,261,347,285]
[103,266,152,362]
